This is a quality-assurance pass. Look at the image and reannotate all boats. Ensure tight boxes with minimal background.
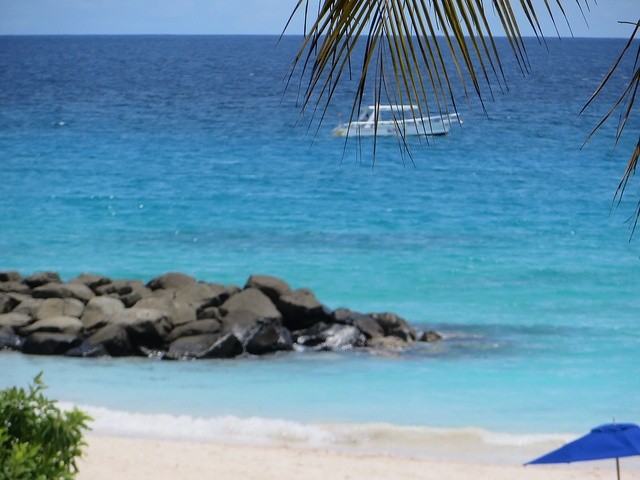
[331,104,464,138]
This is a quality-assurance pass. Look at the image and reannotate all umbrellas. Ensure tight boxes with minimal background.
[524,418,640,479]
